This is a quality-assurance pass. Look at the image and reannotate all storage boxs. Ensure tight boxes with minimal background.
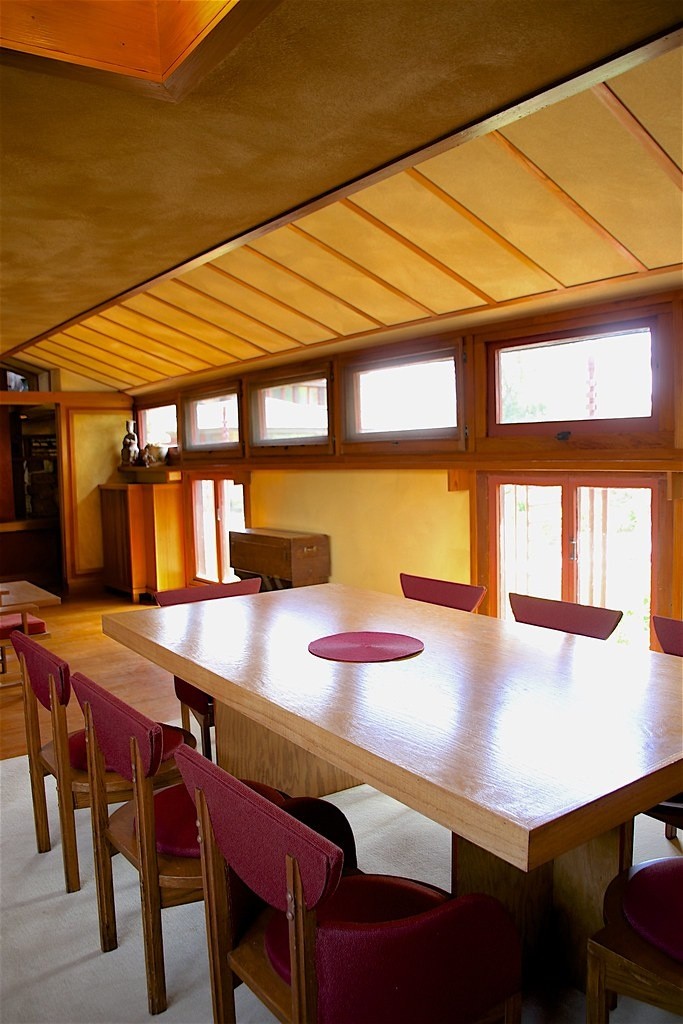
[229,527,332,581]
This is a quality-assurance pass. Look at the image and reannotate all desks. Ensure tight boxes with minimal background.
[0,580,61,609]
[101,584,683,1009]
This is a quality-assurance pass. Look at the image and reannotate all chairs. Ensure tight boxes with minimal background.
[0,571,683,1024]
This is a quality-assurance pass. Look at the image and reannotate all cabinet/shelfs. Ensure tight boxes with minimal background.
[100,483,186,604]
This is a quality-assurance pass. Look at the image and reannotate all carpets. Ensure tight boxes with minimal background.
[0,714,683,1024]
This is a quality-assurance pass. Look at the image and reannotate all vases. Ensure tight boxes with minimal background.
[147,446,168,468]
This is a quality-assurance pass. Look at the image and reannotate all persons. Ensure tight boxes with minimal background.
[121,420,139,466]
